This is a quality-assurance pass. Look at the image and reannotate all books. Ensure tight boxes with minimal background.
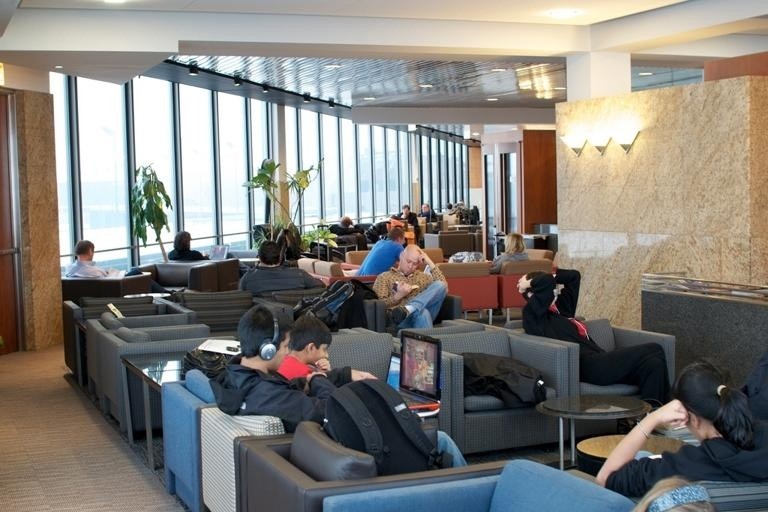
[393,281,420,299]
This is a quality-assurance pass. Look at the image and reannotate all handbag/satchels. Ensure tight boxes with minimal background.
[180,346,233,380]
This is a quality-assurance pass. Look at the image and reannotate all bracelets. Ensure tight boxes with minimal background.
[636,422,649,440]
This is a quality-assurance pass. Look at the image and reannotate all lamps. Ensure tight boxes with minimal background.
[565,135,587,156]
[615,131,641,154]
[590,136,611,156]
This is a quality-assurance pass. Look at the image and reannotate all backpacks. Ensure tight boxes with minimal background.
[293,280,354,332]
[322,378,453,477]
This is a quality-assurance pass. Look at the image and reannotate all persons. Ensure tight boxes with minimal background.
[516,269,670,406]
[276,314,331,381]
[595,358,768,498]
[372,244,448,328]
[329,202,480,242]
[209,304,467,468]
[355,225,404,276]
[490,232,528,274]
[66,232,326,294]
[632,474,715,512]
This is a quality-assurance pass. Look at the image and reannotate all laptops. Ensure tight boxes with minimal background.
[385,350,400,388]
[210,244,228,261]
[393,329,443,420]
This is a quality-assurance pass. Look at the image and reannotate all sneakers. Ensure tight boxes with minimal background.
[386,306,406,324]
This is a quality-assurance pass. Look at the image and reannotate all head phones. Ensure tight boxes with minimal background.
[256,303,283,361]
